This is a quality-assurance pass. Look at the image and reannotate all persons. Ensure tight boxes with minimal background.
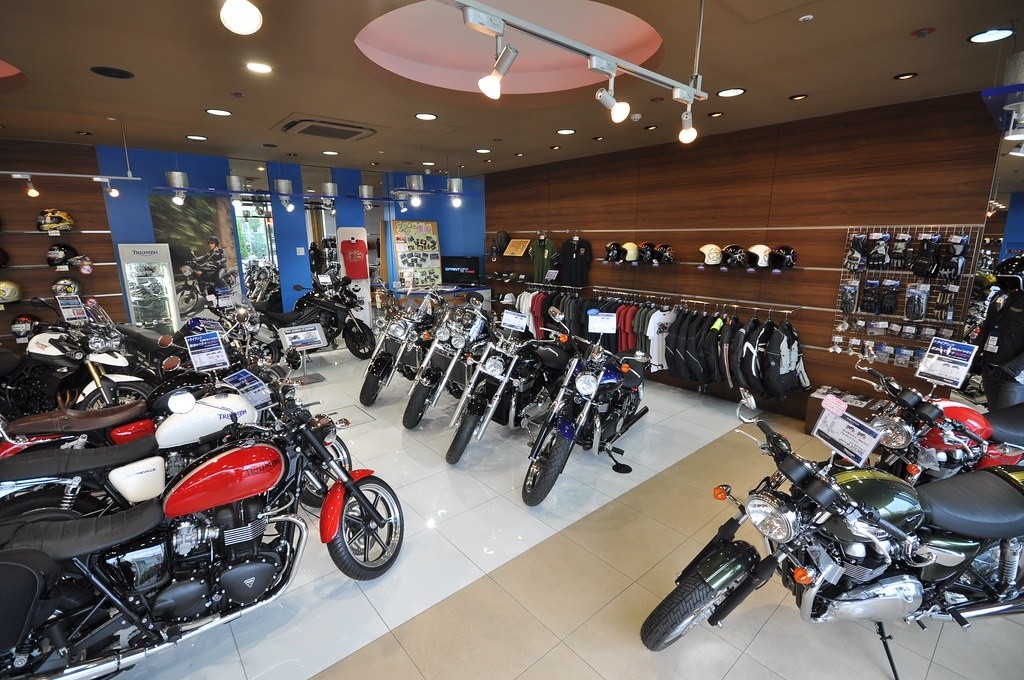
[193,236,228,296]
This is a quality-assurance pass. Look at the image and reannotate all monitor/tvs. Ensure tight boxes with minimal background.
[441,255,479,286]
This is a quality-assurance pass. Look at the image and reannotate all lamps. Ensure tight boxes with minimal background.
[438,0,707,144]
[13,174,39,198]
[92,177,120,198]
[166,150,463,212]
[1003,50,1024,157]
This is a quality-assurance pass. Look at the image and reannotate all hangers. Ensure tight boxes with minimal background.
[524,282,793,332]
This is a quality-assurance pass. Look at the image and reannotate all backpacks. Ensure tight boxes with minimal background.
[490,231,511,256]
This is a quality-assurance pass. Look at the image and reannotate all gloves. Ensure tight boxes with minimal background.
[844,232,911,272]
[840,285,857,312]
[912,235,940,277]
[861,282,879,313]
[934,285,957,321]
[906,295,923,320]
[940,235,968,280]
[876,289,898,316]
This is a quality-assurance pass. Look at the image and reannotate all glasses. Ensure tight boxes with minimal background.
[209,242,215,244]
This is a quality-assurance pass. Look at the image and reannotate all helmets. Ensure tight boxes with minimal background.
[47,244,78,267]
[699,244,797,269]
[11,314,42,337]
[51,277,81,296]
[604,241,674,264]
[208,236,220,246]
[310,242,317,248]
[994,255,1024,290]
[0,249,8,269]
[36,208,74,231]
[0,280,21,303]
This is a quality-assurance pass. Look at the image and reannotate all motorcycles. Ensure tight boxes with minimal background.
[0,260,652,680]
[175,250,240,316]
[851,364,1024,489]
[640,388,1023,680]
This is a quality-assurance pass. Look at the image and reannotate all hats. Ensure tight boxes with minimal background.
[488,271,528,282]
[489,293,516,305]
